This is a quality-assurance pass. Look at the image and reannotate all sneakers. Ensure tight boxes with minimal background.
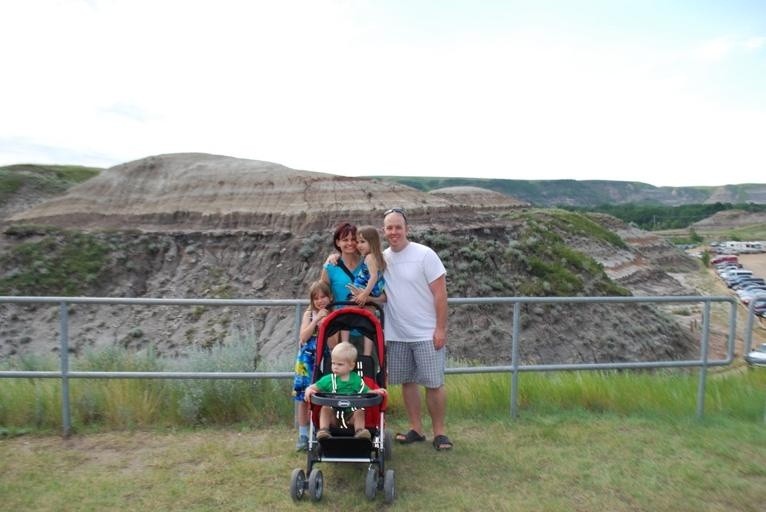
[296,435,309,452]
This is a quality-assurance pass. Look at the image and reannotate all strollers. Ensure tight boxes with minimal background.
[289,300,397,506]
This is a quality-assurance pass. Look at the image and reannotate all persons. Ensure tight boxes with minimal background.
[291,209,453,450]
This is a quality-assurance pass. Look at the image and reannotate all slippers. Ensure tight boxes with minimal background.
[432,435,453,450]
[395,430,426,443]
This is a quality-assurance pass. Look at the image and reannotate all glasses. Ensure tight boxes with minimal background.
[384,207,403,217]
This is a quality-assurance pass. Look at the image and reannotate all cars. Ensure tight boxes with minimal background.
[710,254,766,367]
